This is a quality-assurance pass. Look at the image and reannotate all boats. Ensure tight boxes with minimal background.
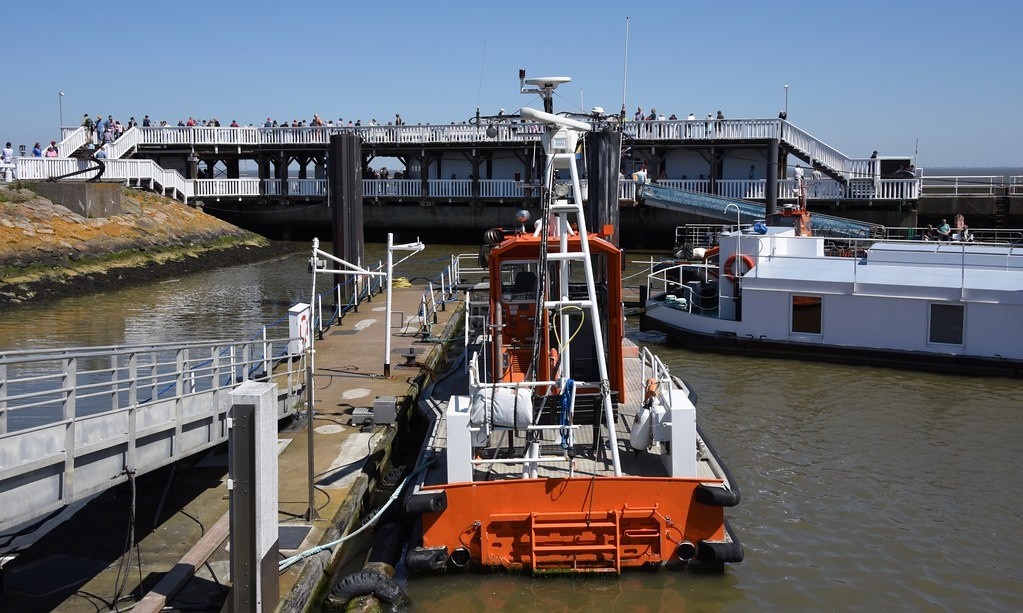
[640,158,1023,380]
[405,15,746,574]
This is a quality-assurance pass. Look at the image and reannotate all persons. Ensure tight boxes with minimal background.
[619,165,821,197]
[778,110,787,137]
[923,218,973,241]
[633,107,724,137]
[0,114,407,185]
[868,151,914,177]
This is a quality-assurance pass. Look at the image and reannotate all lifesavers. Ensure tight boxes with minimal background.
[724,254,754,285]
[550,348,559,395]
[321,570,412,613]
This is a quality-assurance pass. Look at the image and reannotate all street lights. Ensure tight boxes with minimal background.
[784,83,788,120]
[59,92,64,126]
